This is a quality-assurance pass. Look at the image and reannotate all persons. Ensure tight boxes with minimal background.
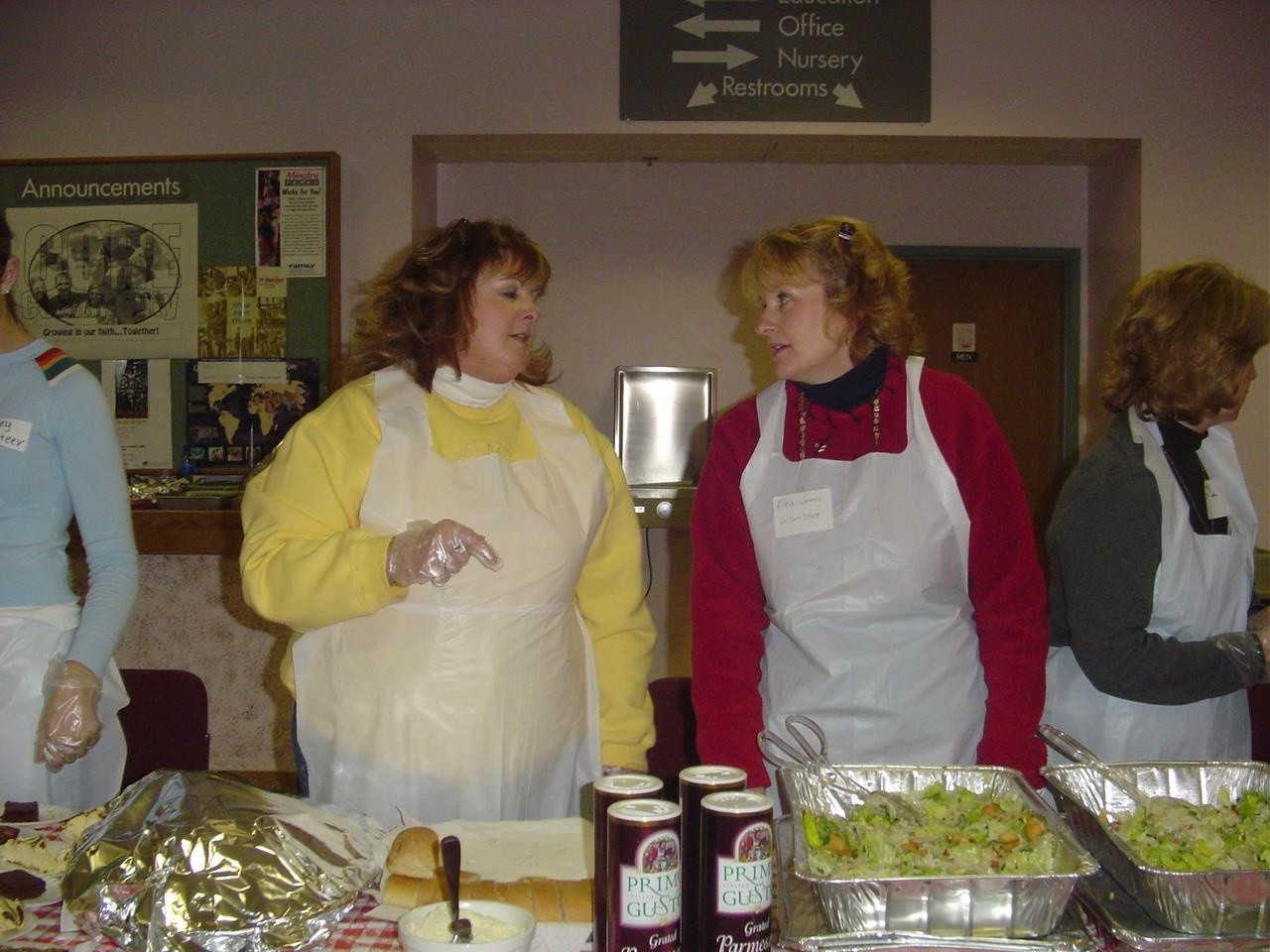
[0,229,139,815]
[237,216,660,832]
[1039,258,1270,775]
[686,213,1049,822]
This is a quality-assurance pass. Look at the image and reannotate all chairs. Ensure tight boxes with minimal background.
[119,669,210,790]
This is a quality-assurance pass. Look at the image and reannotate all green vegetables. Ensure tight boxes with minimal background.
[803,779,1050,876]
[1100,787,1270,872]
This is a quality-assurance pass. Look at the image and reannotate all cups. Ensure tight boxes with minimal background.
[697,790,774,952]
[592,773,665,951]
[607,798,683,951]
[679,764,749,951]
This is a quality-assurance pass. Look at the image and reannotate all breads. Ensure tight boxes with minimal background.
[0,801,39,823]
[382,827,595,923]
[0,869,46,900]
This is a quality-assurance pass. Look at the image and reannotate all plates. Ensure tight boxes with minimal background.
[0,803,77,827]
[0,868,62,906]
[0,827,42,844]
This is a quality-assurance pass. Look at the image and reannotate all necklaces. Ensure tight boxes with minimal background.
[1157,434,1220,538]
[794,389,884,463]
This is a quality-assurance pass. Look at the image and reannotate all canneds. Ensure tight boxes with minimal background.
[606,799,683,952]
[701,792,774,952]
[679,765,747,952]
[593,774,664,952]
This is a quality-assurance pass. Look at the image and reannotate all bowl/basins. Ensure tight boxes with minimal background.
[397,899,536,952]
